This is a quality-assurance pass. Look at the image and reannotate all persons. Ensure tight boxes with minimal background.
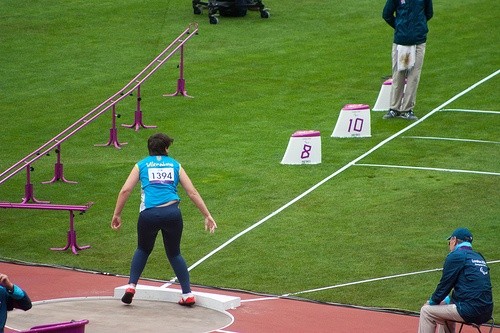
[0,274,32,333]
[381,0,434,120]
[419,228,494,333]
[111,134,218,306]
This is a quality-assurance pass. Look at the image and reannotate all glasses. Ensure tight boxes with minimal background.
[448,240,451,245]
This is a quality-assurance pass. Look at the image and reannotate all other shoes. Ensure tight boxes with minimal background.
[122,288,135,304]
[178,295,195,305]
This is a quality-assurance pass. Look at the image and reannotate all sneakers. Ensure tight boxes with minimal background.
[405,110,417,119]
[383,109,401,118]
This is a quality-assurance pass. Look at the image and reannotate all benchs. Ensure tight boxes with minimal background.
[444,320,500,333]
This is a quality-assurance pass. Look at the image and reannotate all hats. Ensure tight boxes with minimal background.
[446,227,473,244]
